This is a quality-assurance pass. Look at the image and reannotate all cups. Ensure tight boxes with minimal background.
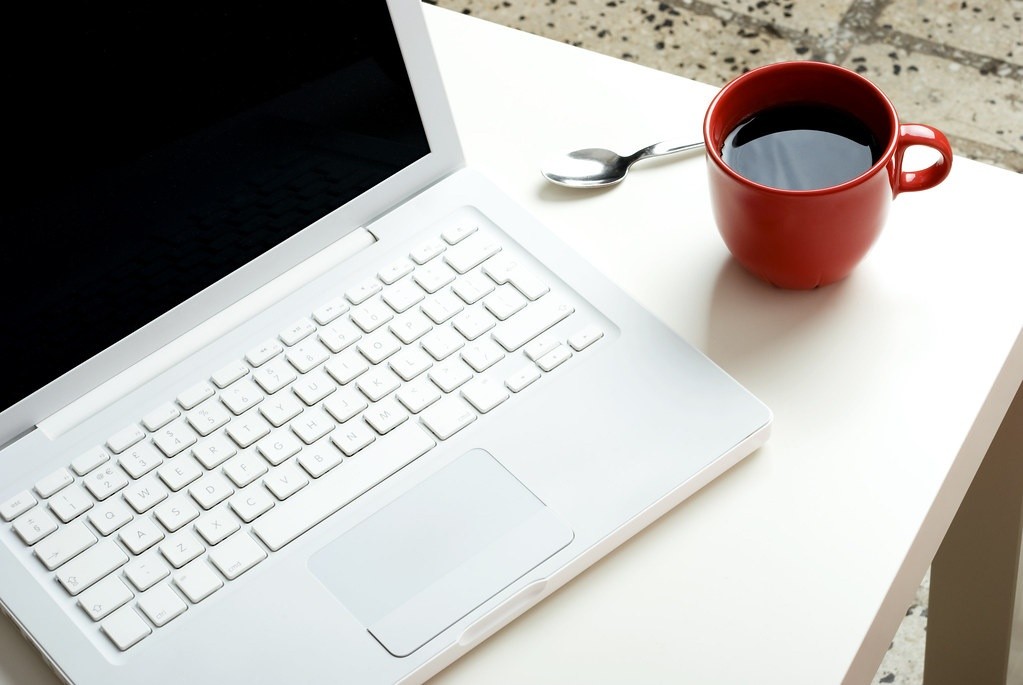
[703,60,952,291]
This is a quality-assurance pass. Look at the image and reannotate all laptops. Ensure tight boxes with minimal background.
[0,0,774,685]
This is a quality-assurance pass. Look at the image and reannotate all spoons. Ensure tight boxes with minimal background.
[540,135,706,189]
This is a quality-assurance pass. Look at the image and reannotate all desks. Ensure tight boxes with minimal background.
[0,0,1023,685]
